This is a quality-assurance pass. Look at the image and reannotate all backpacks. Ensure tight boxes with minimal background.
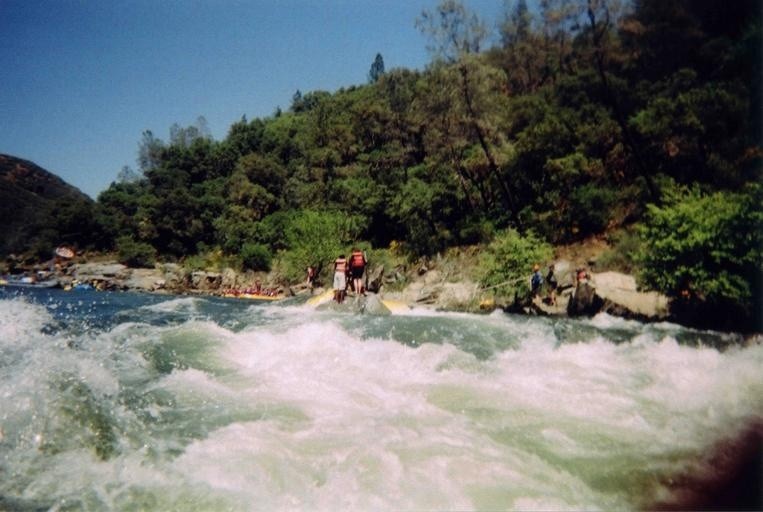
[352,252,365,267]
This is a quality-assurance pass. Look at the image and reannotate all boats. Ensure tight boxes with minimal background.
[64,280,102,292]
[0,275,59,289]
[306,288,408,313]
[225,293,284,300]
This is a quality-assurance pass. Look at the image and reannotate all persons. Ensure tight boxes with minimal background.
[332,246,367,303]
[531,265,594,316]
[306,266,316,294]
